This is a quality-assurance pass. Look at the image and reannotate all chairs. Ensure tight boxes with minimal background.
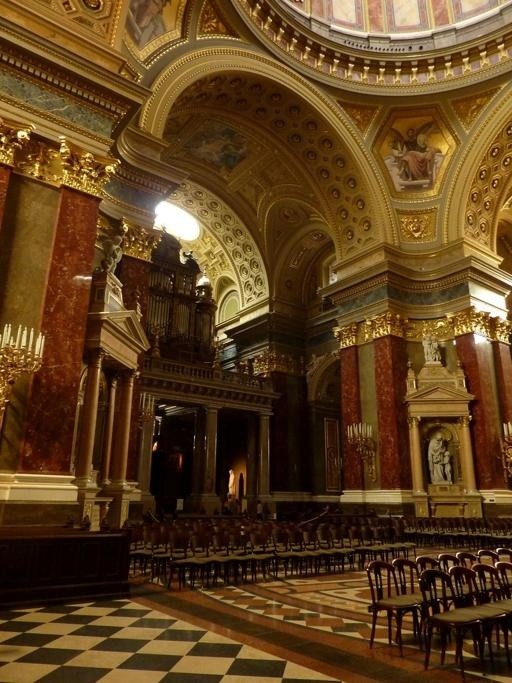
[366,513,512,682]
[124,508,421,589]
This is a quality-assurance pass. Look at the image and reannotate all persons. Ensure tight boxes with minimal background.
[440,452,452,484]
[104,235,122,273]
[423,334,442,361]
[428,434,445,483]
[386,129,441,192]
